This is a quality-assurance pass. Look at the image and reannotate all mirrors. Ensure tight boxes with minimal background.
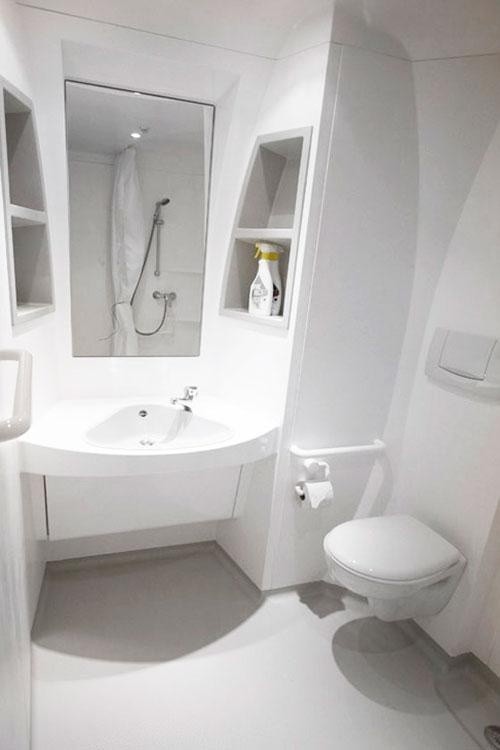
[62,77,215,358]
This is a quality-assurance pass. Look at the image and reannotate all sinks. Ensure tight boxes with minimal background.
[83,403,233,450]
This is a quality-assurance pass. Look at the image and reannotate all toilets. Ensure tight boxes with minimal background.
[322,516,468,620]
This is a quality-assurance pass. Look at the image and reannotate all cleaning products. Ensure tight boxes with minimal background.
[248,241,278,316]
[269,243,286,316]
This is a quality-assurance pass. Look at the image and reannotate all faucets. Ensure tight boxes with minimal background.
[171,387,199,410]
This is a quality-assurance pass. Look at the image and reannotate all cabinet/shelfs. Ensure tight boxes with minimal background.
[220,126,311,331]
[0,74,57,328]
